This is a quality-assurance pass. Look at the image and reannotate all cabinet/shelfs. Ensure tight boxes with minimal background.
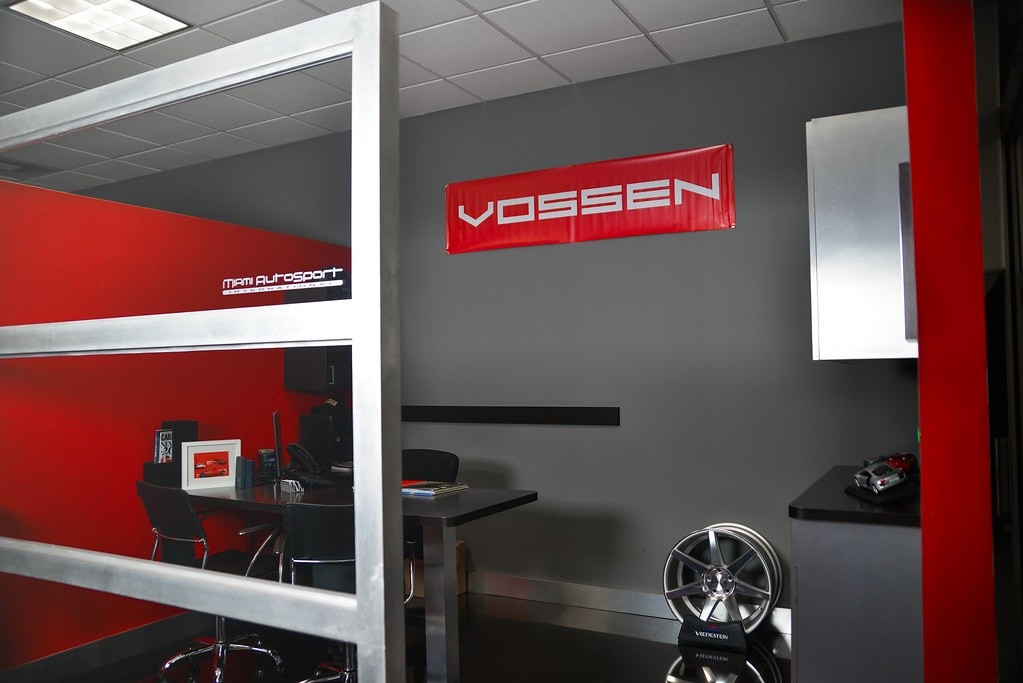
[791,463,925,683]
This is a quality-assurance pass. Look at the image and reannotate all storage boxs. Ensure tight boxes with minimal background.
[403,540,467,599]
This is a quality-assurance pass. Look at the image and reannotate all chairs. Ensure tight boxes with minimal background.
[135,480,282,683]
[404,449,460,606]
[279,501,415,683]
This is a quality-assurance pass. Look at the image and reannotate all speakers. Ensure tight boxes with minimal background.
[297,414,353,471]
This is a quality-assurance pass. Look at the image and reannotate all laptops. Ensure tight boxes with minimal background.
[271,410,339,488]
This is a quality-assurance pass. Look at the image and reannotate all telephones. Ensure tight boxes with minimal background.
[283,442,322,478]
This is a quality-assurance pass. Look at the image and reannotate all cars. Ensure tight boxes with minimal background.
[863,453,917,474]
[854,461,906,493]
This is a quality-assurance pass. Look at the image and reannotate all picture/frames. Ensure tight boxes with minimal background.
[182,439,242,491]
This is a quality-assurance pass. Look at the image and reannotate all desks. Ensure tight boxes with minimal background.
[187,480,537,683]
